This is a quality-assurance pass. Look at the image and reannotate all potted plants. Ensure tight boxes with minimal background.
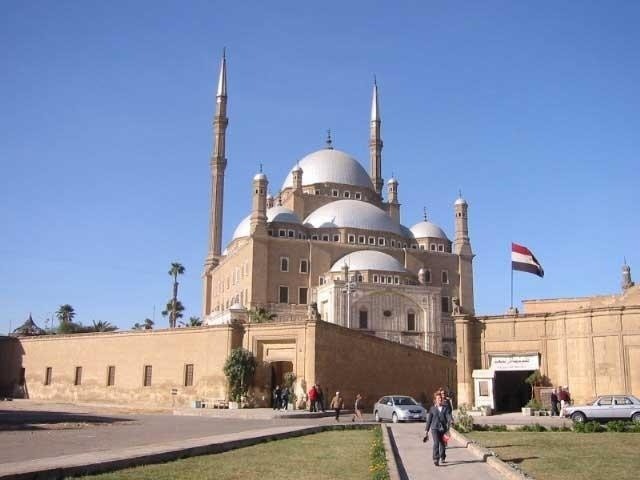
[287,394,296,409]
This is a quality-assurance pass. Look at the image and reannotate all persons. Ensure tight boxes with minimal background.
[273,385,290,410]
[550,386,570,416]
[331,392,344,420]
[352,394,362,422]
[309,385,325,412]
[423,387,453,466]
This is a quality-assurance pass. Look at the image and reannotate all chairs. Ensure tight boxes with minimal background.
[395,399,407,404]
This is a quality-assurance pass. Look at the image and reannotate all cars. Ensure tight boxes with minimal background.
[563,394,640,423]
[373,395,427,423]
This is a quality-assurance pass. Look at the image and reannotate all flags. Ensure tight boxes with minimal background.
[511,242,544,278]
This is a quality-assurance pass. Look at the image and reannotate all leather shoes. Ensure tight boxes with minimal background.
[433,458,445,466]
